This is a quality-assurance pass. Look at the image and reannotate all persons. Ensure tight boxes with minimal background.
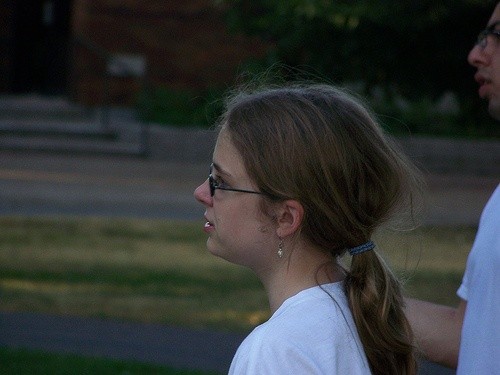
[194,81,417,375]
[402,0,500,375]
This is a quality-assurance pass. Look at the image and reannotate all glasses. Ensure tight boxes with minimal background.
[209,163,266,197]
[478,30,500,48]
